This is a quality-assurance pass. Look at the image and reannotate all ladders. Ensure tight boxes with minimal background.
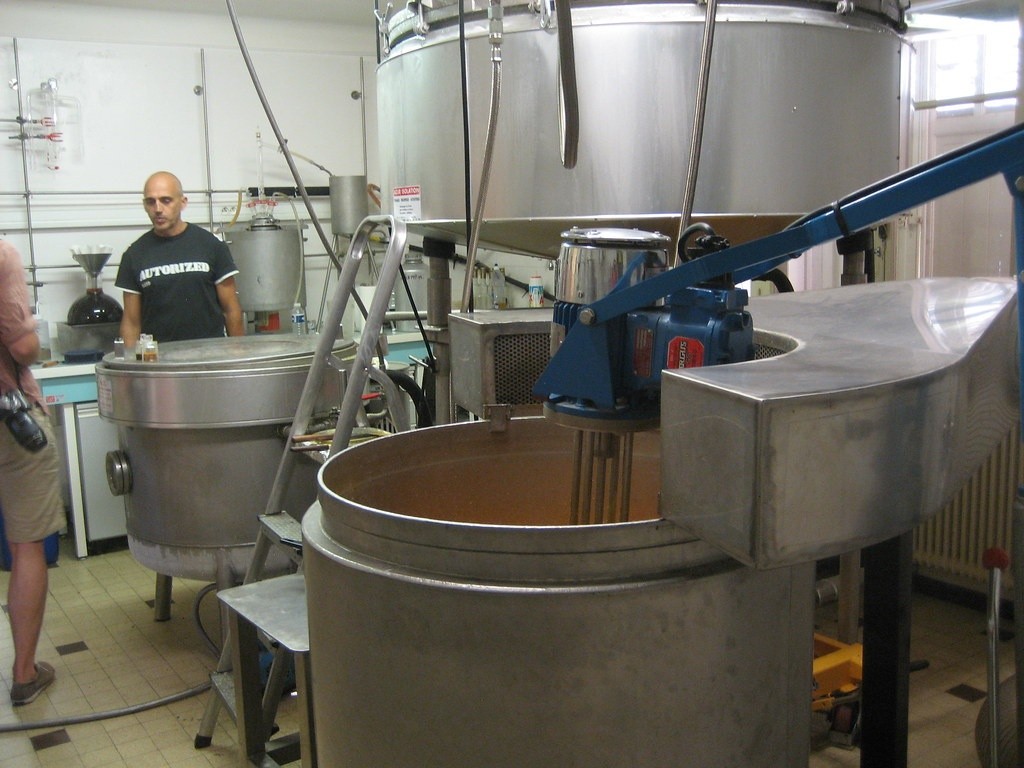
[192,215,409,747]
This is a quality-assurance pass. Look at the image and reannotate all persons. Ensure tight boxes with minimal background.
[114,171,243,346]
[0,239,67,706]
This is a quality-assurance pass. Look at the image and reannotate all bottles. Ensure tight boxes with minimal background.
[135,333,159,363]
[473,264,512,310]
[291,302,307,338]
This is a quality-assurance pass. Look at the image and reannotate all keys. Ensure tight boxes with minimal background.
[0,388,48,453]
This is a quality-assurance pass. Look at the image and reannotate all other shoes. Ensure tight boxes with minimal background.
[10,662,55,705]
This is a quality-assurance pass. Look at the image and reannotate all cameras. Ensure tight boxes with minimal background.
[0,387,47,453]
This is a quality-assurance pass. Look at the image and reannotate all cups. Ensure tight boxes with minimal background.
[529,276,545,308]
[113,336,126,360]
